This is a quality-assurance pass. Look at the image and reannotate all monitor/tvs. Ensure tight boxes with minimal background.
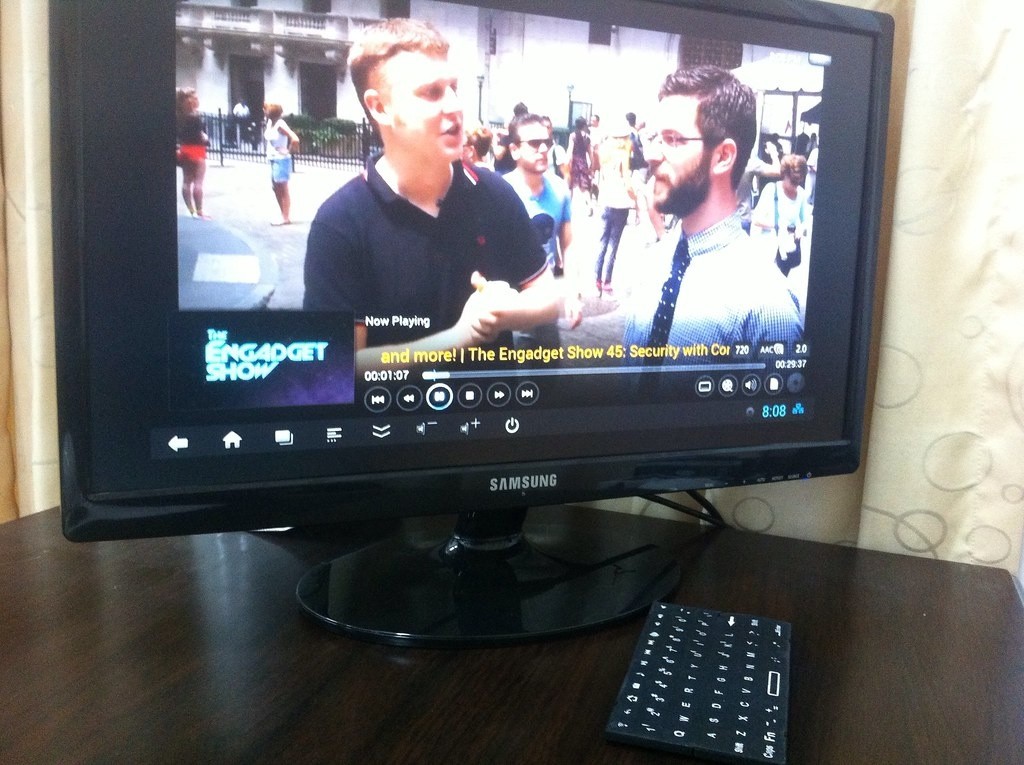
[47,0,896,640]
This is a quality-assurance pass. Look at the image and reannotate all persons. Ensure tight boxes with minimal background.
[261,101,300,226]
[302,16,819,368]
[173,86,209,219]
[233,98,250,118]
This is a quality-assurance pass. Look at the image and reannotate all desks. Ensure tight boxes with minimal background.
[0,506,1023,765]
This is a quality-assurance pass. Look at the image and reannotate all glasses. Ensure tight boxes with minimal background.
[643,130,709,148]
[515,138,553,149]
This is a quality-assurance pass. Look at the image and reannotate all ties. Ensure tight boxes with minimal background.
[639,236,691,392]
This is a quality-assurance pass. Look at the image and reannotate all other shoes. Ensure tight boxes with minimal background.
[596,279,614,292]
[271,219,290,226]
[191,210,212,221]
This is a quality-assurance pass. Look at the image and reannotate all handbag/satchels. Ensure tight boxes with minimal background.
[777,236,800,268]
